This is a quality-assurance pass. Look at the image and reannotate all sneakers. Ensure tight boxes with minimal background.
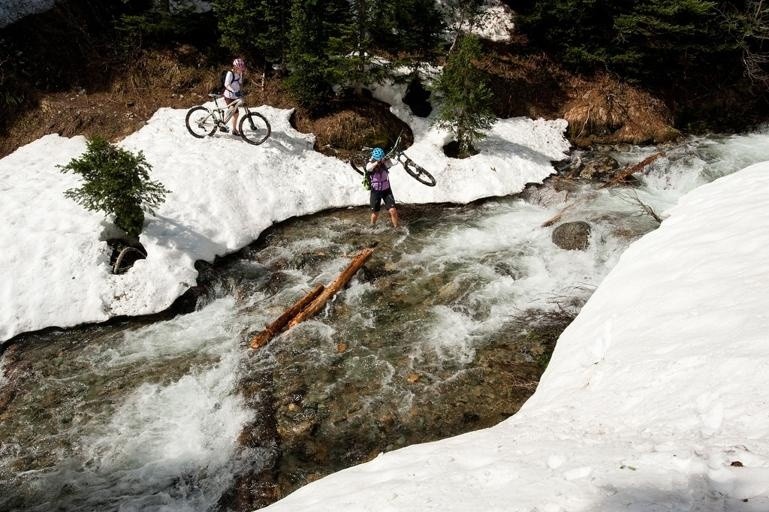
[232,129,240,136]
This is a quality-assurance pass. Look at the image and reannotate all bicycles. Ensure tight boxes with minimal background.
[351,129,436,186]
[186,90,270,145]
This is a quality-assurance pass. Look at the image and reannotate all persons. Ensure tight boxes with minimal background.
[218,57,246,136]
[366,147,402,230]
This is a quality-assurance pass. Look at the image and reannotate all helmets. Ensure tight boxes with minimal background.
[371,147,385,161]
[233,58,246,71]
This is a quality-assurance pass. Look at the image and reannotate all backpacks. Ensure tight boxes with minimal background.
[362,166,371,190]
[220,69,235,87]
